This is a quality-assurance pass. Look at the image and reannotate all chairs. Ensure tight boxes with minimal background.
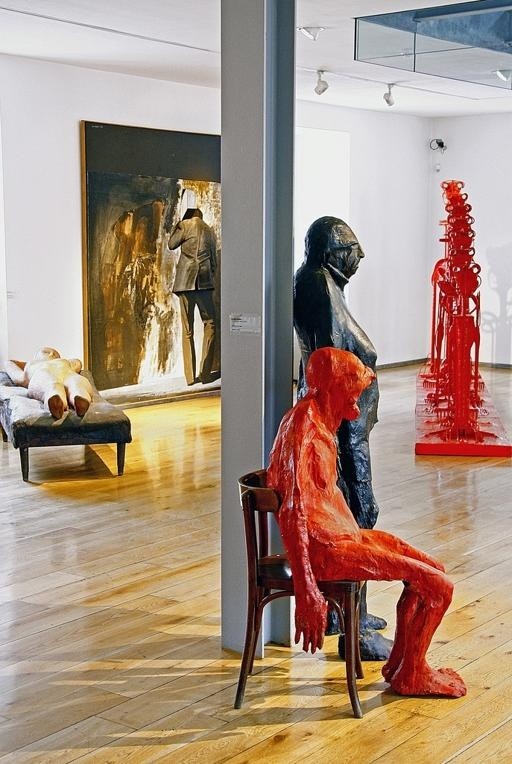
[227,471,368,719]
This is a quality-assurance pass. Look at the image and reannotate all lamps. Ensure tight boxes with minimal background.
[311,68,332,98]
[382,82,397,109]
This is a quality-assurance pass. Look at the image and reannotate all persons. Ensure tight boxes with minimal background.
[2,344,92,421]
[267,346,469,702]
[167,206,220,387]
[293,215,397,663]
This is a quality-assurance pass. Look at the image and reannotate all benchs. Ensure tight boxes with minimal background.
[0,370,134,481]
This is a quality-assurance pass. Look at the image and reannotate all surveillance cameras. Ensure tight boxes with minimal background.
[436,139,445,149]
